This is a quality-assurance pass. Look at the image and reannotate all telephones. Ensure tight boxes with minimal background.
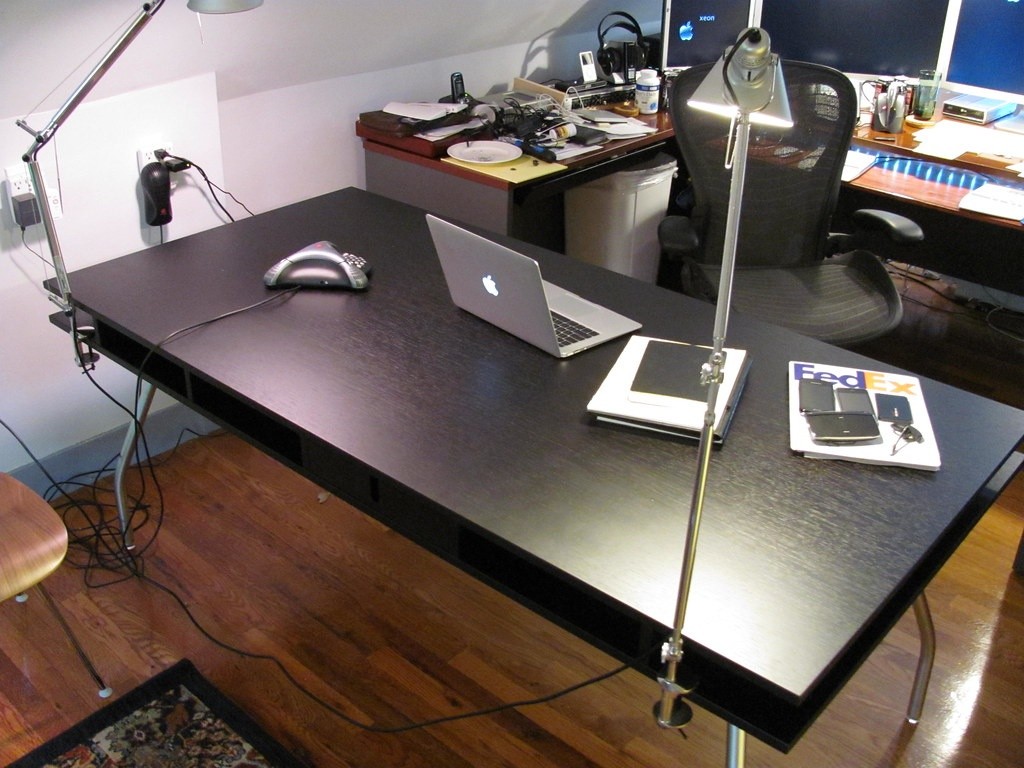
[440,72,478,112]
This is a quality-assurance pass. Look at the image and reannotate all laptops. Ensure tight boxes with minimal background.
[426,214,642,359]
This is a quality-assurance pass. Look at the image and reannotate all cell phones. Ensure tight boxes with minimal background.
[800,378,835,412]
[836,388,874,414]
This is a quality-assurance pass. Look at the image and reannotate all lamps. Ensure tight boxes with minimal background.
[23,0,265,367]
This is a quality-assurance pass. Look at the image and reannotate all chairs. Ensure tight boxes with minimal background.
[0,470,116,698]
[654,59,926,346]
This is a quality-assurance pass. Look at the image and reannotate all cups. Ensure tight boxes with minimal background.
[914,69,942,121]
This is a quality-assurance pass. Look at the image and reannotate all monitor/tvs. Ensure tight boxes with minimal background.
[752,0,954,87]
[661,0,764,74]
[940,0,1024,105]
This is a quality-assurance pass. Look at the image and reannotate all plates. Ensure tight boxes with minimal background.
[447,141,522,163]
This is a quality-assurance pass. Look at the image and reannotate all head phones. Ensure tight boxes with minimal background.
[596,11,649,76]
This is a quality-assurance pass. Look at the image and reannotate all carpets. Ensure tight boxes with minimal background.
[3,655,312,768]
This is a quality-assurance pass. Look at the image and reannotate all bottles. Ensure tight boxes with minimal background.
[549,123,577,141]
[634,68,660,114]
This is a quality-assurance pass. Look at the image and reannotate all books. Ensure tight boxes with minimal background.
[581,109,629,122]
[586,334,755,450]
[788,359,941,473]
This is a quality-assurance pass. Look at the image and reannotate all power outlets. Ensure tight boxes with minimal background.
[135,139,180,192]
[5,161,60,227]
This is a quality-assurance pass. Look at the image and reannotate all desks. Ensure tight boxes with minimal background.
[355,71,1024,301]
[43,185,1024,768]
